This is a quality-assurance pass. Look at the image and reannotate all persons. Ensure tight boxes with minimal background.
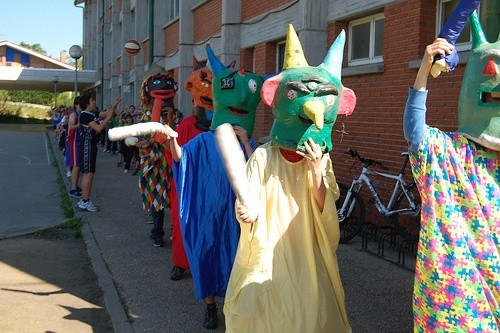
[223,66,353,333]
[65,95,83,199]
[152,69,213,281]
[77,95,121,211]
[403,38,500,333]
[164,69,265,329]
[98,104,145,176]
[134,72,183,246]
[52,105,73,155]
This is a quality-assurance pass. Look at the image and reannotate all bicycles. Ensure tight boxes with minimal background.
[334,148,423,244]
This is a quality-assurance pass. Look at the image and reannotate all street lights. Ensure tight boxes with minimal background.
[69,45,85,98]
[51,74,59,106]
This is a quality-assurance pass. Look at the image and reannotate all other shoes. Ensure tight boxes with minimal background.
[132,168,138,175]
[146,213,154,223]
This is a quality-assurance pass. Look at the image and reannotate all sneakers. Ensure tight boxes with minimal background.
[69,190,82,198]
[125,169,128,173]
[205,302,218,329]
[154,240,160,246]
[70,186,82,193]
[170,265,185,280]
[78,199,98,211]
[104,149,110,152]
[67,168,72,176]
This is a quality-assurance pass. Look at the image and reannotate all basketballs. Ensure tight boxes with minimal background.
[124,40,141,56]
